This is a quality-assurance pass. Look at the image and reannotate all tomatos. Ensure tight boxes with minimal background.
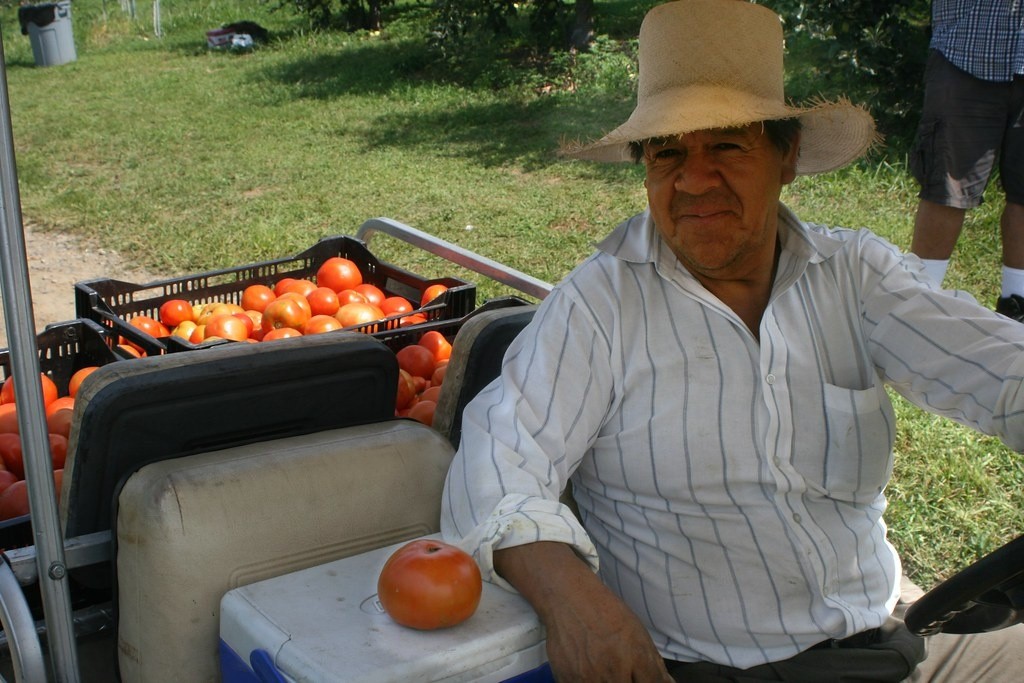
[0,366,101,521]
[104,258,453,428]
[378,539,482,630]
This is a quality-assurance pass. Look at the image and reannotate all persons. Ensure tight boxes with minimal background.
[439,0,1023,683]
[909,0,1024,323]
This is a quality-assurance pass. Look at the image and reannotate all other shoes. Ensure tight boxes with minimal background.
[996,293,1024,323]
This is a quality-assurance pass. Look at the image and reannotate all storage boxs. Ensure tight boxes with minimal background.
[206,28,235,50]
[0,235,556,683]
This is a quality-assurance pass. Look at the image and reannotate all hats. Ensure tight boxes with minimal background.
[557,0,877,177]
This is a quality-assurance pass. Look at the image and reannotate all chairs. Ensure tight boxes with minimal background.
[432,306,539,453]
[115,421,457,683]
[56,330,400,541]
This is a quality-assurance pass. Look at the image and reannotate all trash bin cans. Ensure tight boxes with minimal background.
[17,1,79,68]
